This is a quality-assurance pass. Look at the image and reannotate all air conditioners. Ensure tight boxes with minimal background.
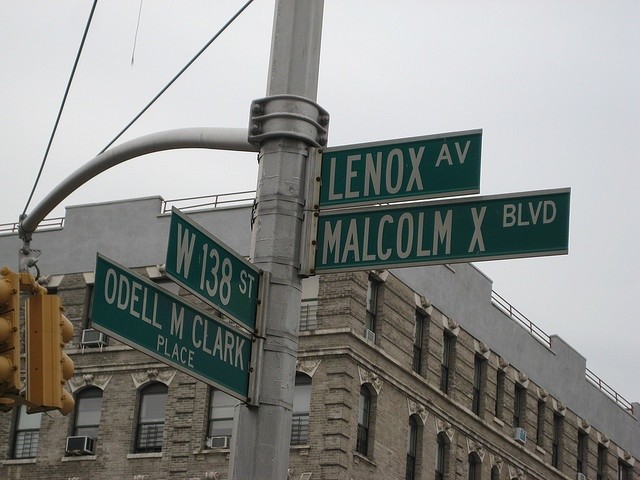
[515,428,529,441]
[81,328,105,345]
[64,436,93,456]
[576,471,586,480]
[210,436,228,448]
[365,328,378,340]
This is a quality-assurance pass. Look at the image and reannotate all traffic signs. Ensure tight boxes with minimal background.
[165,206,261,339]
[319,128,483,210]
[313,186,571,275]
[90,251,252,400]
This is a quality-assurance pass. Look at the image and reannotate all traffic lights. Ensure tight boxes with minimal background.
[59,313,75,416]
[0,266,20,396]
